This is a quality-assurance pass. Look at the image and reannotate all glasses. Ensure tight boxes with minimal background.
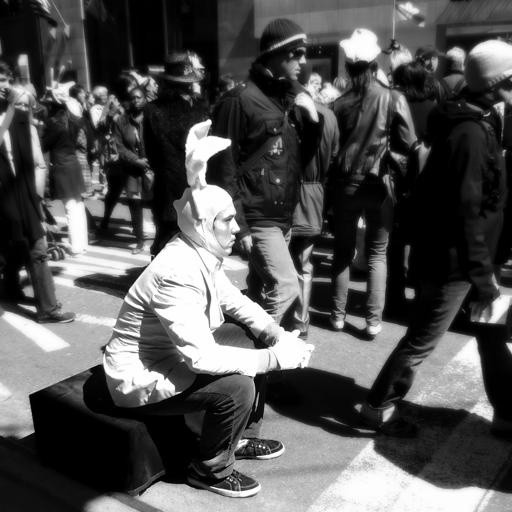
[290,49,308,58]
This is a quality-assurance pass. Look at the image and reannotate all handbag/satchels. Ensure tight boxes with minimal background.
[386,92,412,204]
[144,168,156,191]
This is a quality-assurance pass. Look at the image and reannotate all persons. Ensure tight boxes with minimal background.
[0,59,75,322]
[85,88,108,195]
[285,99,338,340]
[327,28,427,338]
[96,87,154,251]
[32,90,89,256]
[100,119,314,497]
[68,83,88,198]
[208,18,325,326]
[301,70,328,104]
[416,47,467,101]
[143,50,208,261]
[86,92,95,108]
[384,61,445,321]
[96,75,137,133]
[0,84,49,247]
[358,38,512,440]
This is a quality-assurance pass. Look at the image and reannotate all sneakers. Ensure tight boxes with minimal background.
[188,467,260,497]
[233,439,284,460]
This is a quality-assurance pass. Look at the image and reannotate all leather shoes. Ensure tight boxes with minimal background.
[38,311,75,323]
[361,415,413,437]
[492,427,512,438]
[367,323,381,337]
[330,316,345,331]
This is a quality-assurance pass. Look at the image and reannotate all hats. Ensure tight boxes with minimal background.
[446,48,466,72]
[465,41,512,92]
[260,19,307,55]
[415,46,445,62]
[154,54,205,82]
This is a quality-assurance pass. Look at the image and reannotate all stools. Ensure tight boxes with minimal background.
[29,363,206,497]
